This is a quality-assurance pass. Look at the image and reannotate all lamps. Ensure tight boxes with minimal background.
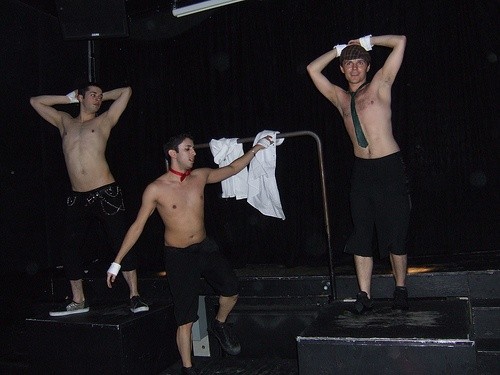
[171,0,245,18]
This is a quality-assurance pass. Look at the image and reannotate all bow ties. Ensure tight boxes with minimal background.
[168,167,191,182]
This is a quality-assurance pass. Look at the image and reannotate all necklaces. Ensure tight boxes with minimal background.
[169,168,192,181]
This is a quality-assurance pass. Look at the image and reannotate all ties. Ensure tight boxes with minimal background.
[348,82,368,149]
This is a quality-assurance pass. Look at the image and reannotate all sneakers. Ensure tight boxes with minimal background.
[209,316,242,356]
[130,296,149,313]
[181,362,208,375]
[392,287,409,311]
[345,291,373,314]
[49,299,89,316]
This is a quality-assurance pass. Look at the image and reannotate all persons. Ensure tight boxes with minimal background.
[28,83,150,316]
[305,35,413,314]
[106,134,275,375]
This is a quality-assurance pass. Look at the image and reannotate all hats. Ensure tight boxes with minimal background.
[339,46,371,67]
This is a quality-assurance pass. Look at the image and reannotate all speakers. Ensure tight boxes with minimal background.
[55,0,130,42]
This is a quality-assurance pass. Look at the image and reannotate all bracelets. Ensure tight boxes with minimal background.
[251,148,256,156]
